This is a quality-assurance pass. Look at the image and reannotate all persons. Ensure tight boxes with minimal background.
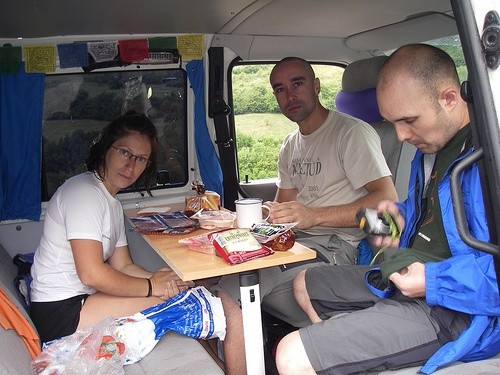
[29,113,196,344]
[213,57,398,375]
[276,44,500,375]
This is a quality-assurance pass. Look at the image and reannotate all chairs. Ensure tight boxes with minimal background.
[0,242,225,375]
[262,55,418,328]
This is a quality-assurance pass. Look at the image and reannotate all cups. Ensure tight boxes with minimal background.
[234,198,271,228]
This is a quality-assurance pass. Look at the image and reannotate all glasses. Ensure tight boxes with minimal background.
[110,145,152,168]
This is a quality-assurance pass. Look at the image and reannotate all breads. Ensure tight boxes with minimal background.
[185,191,220,211]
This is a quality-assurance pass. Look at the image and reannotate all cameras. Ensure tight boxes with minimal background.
[356,207,399,235]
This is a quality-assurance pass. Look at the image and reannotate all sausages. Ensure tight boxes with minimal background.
[253,225,296,251]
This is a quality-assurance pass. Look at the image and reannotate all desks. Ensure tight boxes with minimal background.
[124,202,316,375]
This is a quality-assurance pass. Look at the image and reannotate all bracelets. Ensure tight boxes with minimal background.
[147,279,152,297]
